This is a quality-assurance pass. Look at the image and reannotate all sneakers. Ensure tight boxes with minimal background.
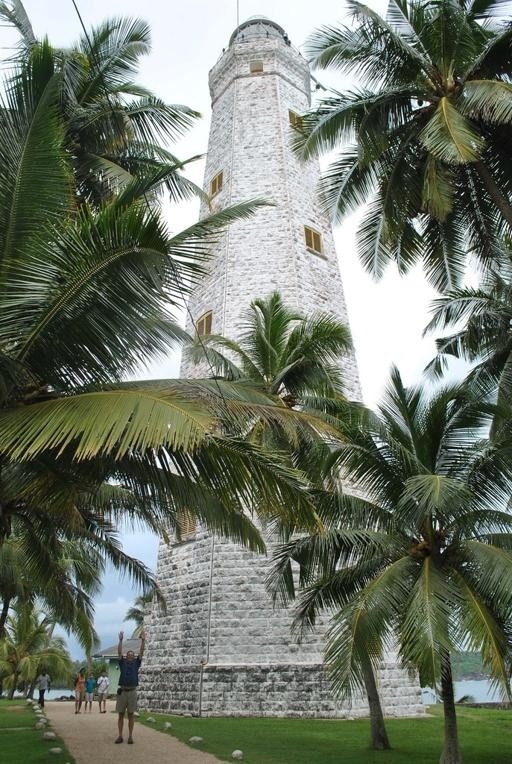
[115,736,134,744]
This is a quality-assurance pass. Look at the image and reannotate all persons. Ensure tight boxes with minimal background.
[116,629,146,744]
[84,672,98,715]
[74,668,86,714]
[97,670,109,713]
[35,668,51,707]
